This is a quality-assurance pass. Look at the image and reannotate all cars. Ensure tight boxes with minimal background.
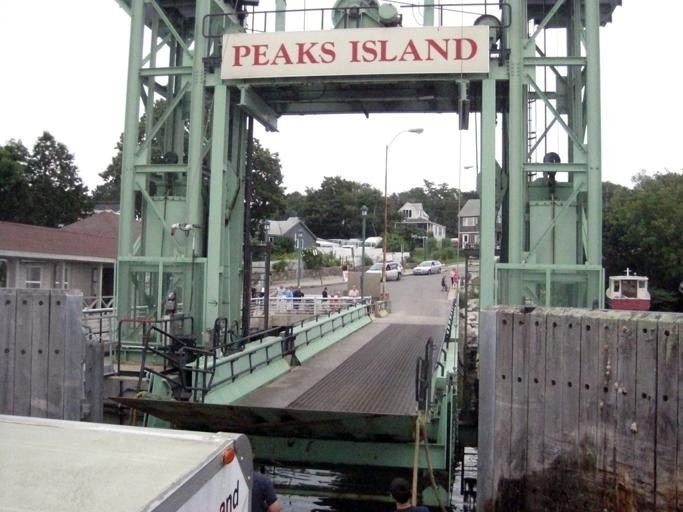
[367,262,403,281]
[413,260,443,276]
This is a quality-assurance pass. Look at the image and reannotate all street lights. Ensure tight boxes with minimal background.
[298,230,304,288]
[382,128,424,298]
[264,222,270,243]
[361,206,368,302]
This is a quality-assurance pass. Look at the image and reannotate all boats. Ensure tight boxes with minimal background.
[606,268,651,310]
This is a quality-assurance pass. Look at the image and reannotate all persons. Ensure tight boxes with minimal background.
[441,267,460,292]
[252,470,281,512]
[250,283,360,313]
[390,477,428,512]
